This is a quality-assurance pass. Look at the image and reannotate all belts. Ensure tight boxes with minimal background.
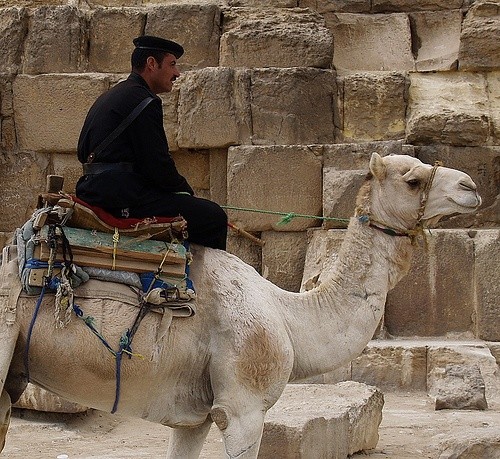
[83,162,125,175]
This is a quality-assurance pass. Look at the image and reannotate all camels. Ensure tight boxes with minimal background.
[0,153,483,459]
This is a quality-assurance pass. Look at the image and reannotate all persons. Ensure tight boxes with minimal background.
[76,36,228,250]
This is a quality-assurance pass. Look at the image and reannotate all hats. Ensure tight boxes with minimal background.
[133,36,184,59]
